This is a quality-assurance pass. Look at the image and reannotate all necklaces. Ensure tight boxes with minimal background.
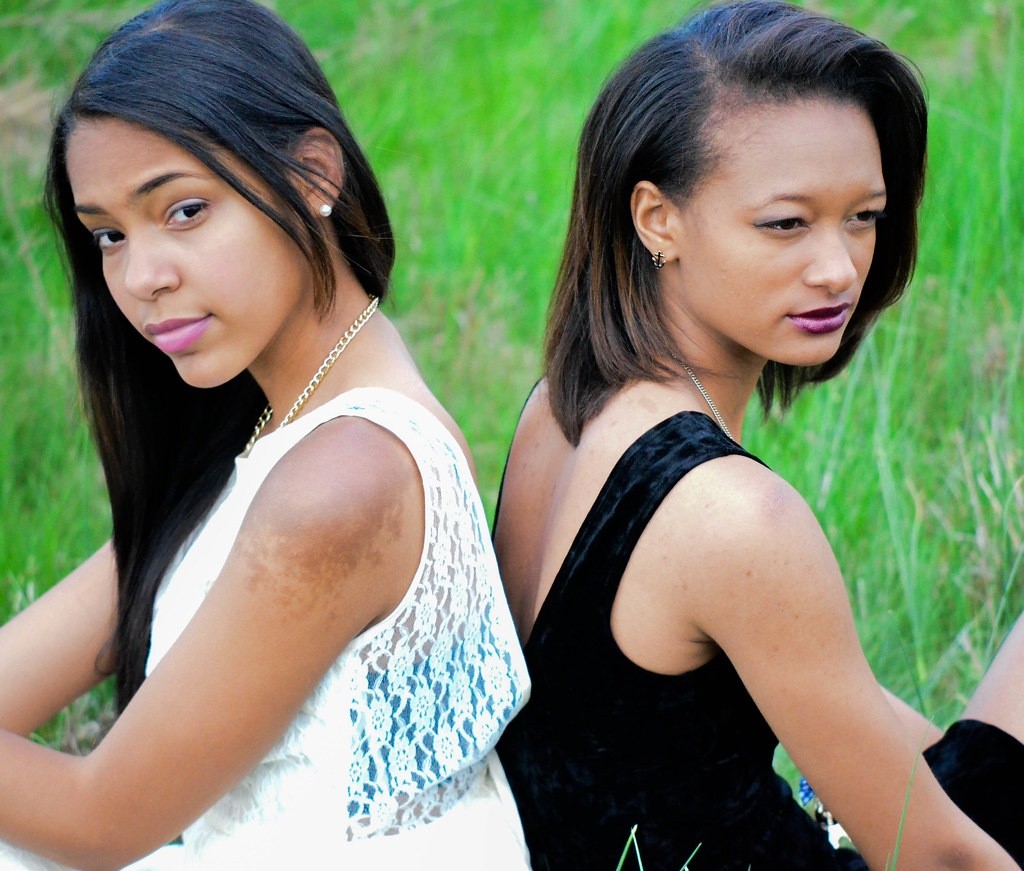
[246,294,380,449]
[673,353,734,441]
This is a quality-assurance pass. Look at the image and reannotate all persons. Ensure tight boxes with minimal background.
[490,1,1024,870]
[1,0,534,871]
